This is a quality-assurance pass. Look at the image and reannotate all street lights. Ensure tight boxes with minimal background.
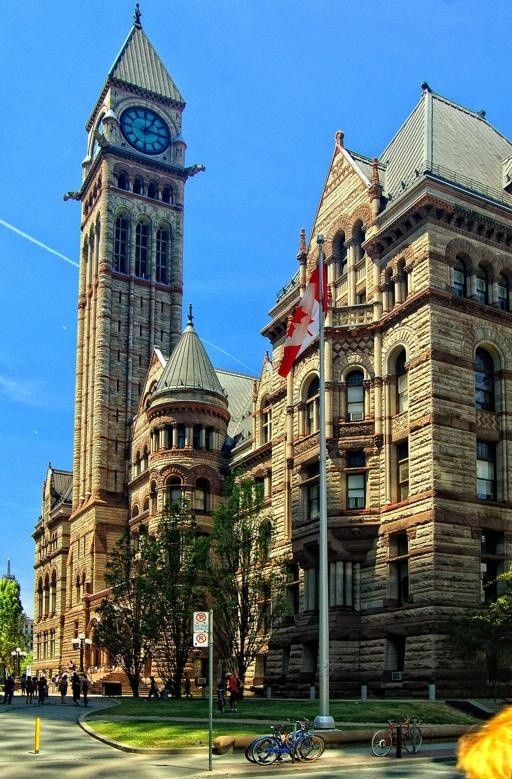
[71,632,93,672]
[10,646,29,676]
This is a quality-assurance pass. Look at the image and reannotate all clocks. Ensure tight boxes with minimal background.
[120,106,171,156]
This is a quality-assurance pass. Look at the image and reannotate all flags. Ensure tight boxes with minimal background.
[276,252,328,378]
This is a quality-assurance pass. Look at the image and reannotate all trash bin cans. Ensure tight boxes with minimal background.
[102,681,122,698]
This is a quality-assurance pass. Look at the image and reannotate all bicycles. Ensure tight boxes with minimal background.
[255,718,322,765]
[369,713,426,758]
[242,717,329,765]
[214,688,227,715]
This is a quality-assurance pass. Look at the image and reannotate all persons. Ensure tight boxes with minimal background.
[143,668,240,713]
[2,658,94,708]
